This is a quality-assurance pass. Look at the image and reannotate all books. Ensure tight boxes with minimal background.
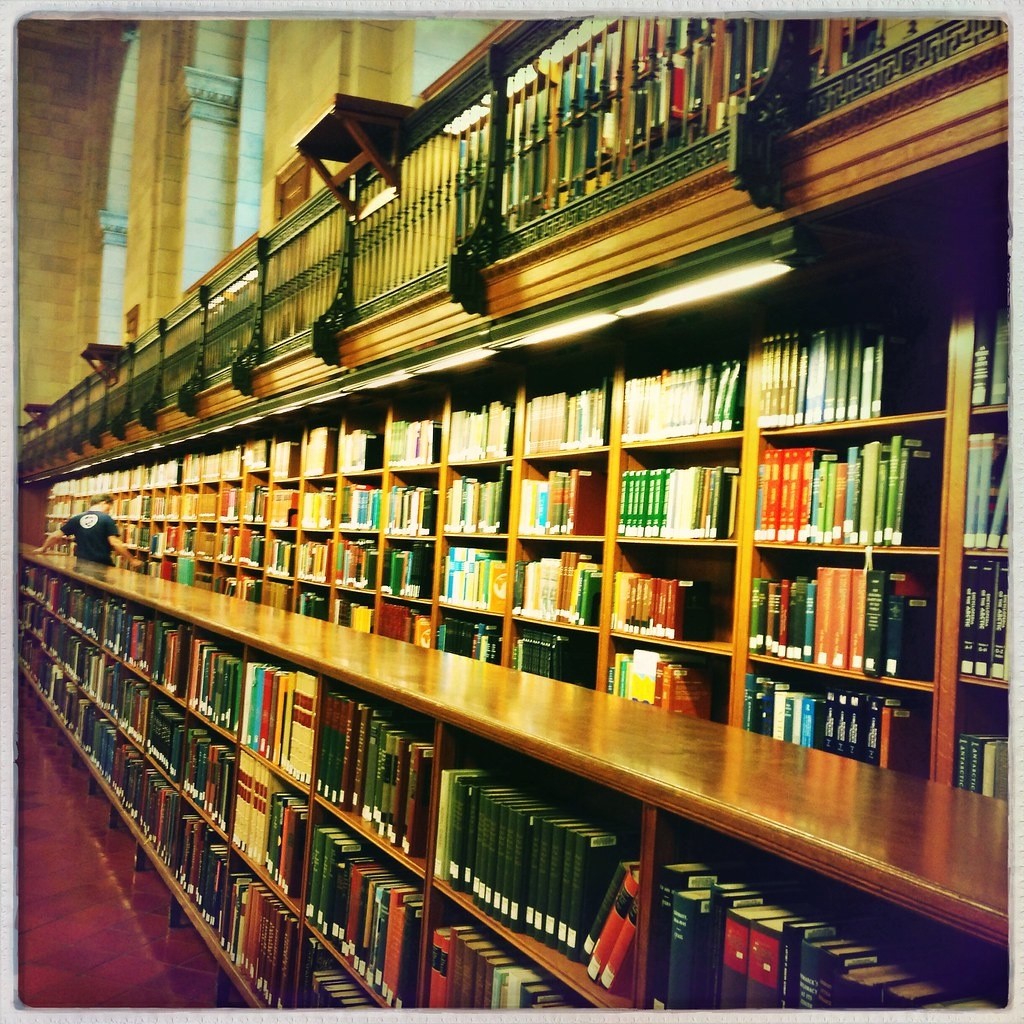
[16,564,190,879]
[180,640,437,1011]
[46,434,266,598]
[261,401,519,665]
[516,308,1010,801]
[427,764,645,1012]
[648,858,1008,1010]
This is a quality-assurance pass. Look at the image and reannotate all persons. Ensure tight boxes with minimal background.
[34,491,149,570]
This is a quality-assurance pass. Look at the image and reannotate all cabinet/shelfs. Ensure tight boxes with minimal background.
[17,19,1010,796]
[17,541,1010,1010]
